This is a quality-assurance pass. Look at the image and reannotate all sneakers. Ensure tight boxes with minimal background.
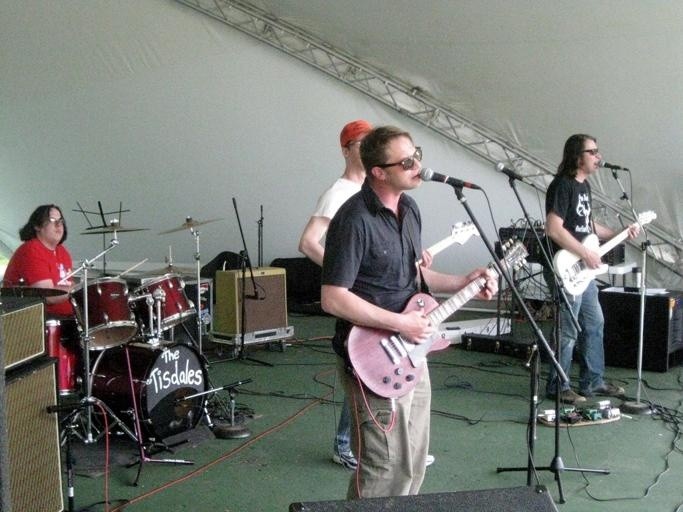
[545,389,587,405]
[591,382,625,395]
[426,455,435,467]
[333,450,358,469]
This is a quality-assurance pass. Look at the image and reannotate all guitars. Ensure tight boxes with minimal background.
[553,210,657,296]
[343,236,530,399]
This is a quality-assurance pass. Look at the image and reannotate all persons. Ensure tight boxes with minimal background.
[2,204,76,318]
[542,132,641,404]
[297,119,436,472]
[318,123,499,499]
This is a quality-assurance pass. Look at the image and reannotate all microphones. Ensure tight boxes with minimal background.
[246,294,259,300]
[495,162,535,187]
[419,167,480,189]
[46,401,94,414]
[598,159,628,171]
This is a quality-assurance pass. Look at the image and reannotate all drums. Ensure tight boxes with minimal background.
[75,336,209,442]
[67,275,139,351]
[130,272,198,339]
[45,319,63,358]
[58,351,78,396]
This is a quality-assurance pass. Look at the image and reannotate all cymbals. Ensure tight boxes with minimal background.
[157,217,224,235]
[80,223,150,234]
[0,285,66,298]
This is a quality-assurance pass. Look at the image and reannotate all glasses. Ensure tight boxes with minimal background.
[44,217,65,225]
[382,147,422,170]
[346,140,362,148]
[581,148,598,155]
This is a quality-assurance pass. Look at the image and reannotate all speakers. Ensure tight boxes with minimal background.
[289,484,559,512]
[212,266,288,337]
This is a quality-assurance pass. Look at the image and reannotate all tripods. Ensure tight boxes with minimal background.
[210,195,275,367]
[60,225,138,447]
[496,181,610,503]
[125,346,193,487]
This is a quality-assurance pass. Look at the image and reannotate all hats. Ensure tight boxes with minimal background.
[340,120,373,147]
